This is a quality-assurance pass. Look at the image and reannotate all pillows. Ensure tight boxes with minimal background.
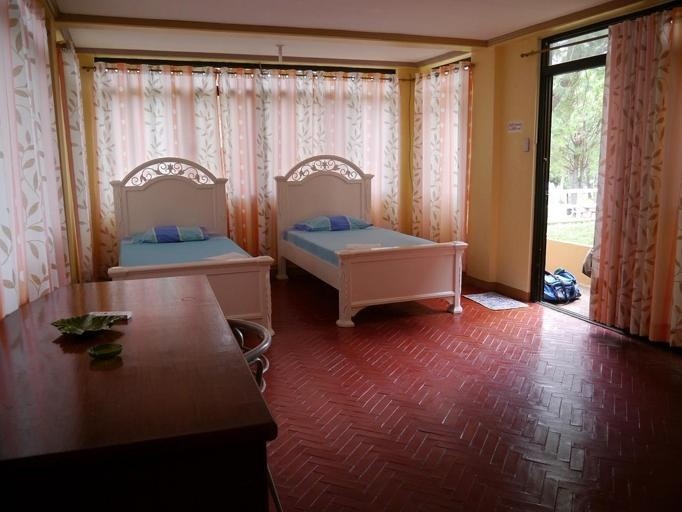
[131,226,209,244]
[293,215,374,232]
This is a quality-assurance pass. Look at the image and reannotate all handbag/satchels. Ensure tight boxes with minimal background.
[543,267,581,303]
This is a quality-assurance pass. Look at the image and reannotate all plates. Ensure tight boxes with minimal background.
[86,343,122,359]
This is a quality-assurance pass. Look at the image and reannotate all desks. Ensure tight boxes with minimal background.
[0,274,278,512]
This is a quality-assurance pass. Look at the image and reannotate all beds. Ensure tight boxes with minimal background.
[274,155,469,328]
[107,158,274,340]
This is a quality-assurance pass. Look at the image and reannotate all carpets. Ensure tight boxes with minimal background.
[461,291,529,310]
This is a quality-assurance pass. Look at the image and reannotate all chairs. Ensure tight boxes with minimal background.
[226,318,283,512]
